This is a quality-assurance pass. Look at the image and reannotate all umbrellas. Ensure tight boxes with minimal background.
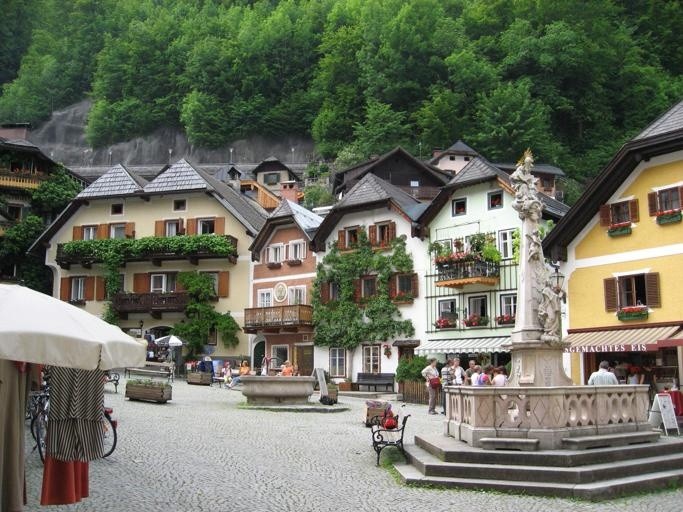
[154,334,190,364]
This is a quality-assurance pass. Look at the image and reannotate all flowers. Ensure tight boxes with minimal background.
[432,312,515,327]
[435,251,480,267]
[383,344,391,352]
[380,404,398,430]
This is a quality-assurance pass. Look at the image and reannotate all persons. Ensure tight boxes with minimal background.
[608,360,658,408]
[420,358,439,414]
[222,362,232,389]
[441,358,507,415]
[225,360,250,388]
[281,360,293,376]
[588,360,618,385]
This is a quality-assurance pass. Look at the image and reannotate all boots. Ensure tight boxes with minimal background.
[429,410,446,415]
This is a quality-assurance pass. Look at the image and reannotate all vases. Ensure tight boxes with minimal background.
[385,352,390,355]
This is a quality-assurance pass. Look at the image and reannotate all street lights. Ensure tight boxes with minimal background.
[139,319,144,337]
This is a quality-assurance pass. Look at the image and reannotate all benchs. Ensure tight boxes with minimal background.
[124,361,174,384]
[212,368,256,388]
[351,372,395,394]
[370,403,412,467]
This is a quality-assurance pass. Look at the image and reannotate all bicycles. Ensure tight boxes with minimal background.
[101,407,118,458]
[30,383,49,465]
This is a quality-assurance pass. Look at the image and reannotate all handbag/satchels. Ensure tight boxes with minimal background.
[430,378,441,389]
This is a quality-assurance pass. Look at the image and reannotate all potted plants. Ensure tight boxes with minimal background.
[326,378,358,399]
[125,379,172,404]
